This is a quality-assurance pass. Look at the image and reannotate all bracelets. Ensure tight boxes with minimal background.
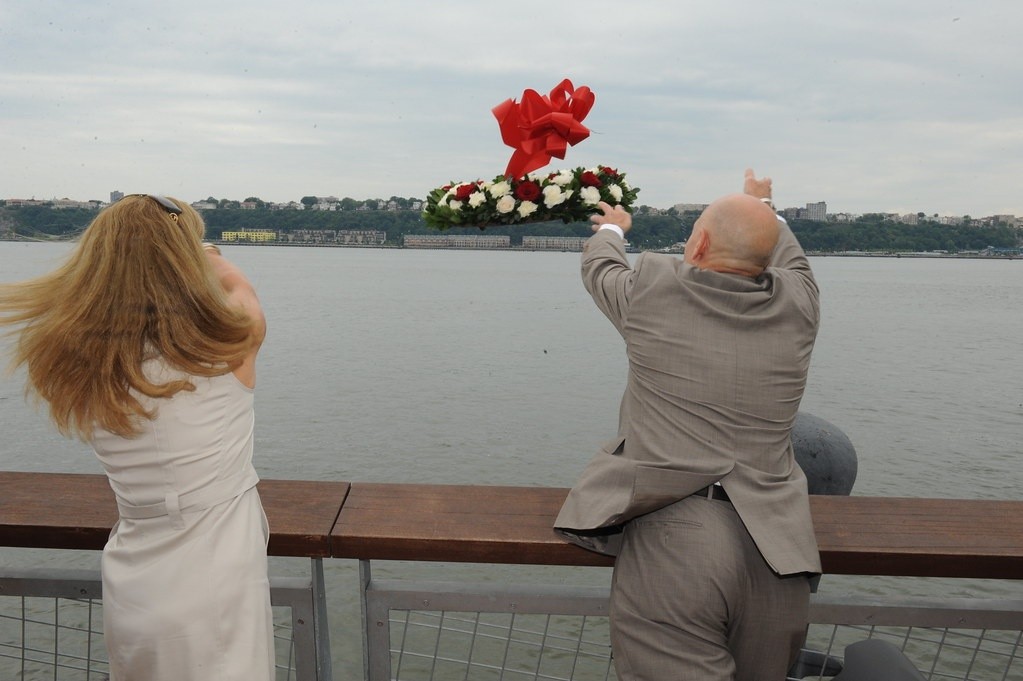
[203,242,220,254]
[761,198,774,209]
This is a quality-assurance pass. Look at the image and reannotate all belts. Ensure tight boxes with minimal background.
[696,483,731,501]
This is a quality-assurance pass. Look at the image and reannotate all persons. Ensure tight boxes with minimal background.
[552,166,822,681]
[0,193,276,681]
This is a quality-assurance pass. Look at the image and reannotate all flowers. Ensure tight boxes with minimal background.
[420,168,641,233]
